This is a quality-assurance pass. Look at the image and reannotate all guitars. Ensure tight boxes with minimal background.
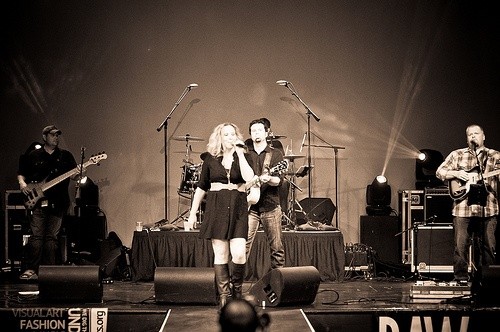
[21,150,108,213]
[448,165,500,201]
[247,161,289,205]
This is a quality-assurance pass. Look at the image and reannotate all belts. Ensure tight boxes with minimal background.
[210,182,246,192]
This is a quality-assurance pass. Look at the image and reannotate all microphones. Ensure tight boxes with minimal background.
[232,142,247,150]
[81,146,86,154]
[470,140,479,147]
[187,84,198,89]
[300,132,307,153]
[276,80,291,85]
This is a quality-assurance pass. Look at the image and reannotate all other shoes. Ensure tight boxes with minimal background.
[21,269,36,279]
[457,280,468,286]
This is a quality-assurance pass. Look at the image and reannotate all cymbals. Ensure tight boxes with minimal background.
[169,135,208,142]
[265,135,287,141]
[171,150,202,155]
[281,153,307,160]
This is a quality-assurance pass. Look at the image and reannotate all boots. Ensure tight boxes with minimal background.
[215,263,231,314]
[229,261,247,301]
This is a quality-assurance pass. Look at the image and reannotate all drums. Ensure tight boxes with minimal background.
[176,167,208,202]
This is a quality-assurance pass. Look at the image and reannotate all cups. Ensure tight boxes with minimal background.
[136,221,143,232]
[184,222,190,231]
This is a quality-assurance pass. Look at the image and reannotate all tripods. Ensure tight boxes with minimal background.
[286,157,314,228]
[395,215,439,282]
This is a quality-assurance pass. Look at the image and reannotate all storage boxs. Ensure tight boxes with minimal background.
[398,188,471,298]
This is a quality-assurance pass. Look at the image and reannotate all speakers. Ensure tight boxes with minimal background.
[38,247,128,304]
[470,265,500,307]
[423,186,455,226]
[155,267,220,306]
[359,215,402,266]
[251,266,321,308]
[289,197,336,225]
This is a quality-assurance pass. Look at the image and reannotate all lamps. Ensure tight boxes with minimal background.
[366,174,393,216]
[415,148,445,190]
[18,142,42,164]
[74,175,100,216]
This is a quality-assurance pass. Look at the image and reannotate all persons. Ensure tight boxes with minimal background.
[435,123,500,283]
[186,124,255,310]
[17,124,87,280]
[245,118,290,226]
[242,120,285,281]
[218,298,258,332]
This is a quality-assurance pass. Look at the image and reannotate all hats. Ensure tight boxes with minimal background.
[42,125,62,136]
